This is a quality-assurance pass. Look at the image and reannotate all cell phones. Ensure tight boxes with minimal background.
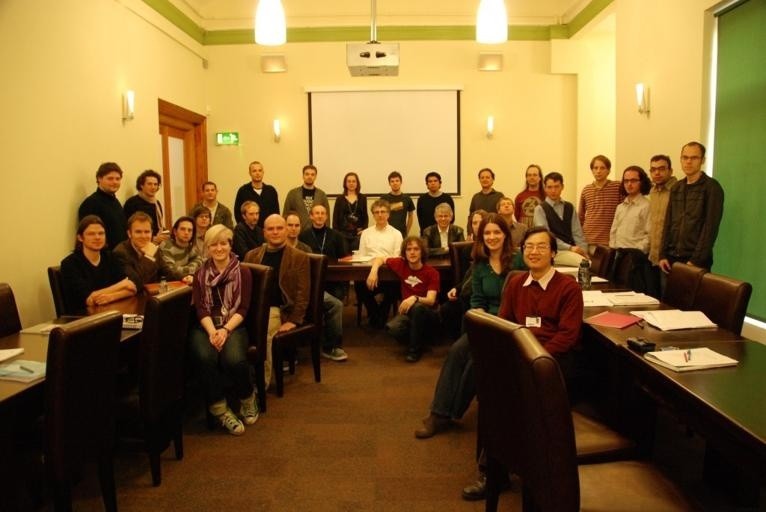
[160,230,170,235]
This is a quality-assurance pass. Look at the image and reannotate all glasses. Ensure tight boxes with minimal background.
[373,211,388,213]
[624,179,640,183]
[682,156,700,160]
[651,168,669,171]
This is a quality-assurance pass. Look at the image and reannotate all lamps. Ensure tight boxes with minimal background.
[475,0,508,44]
[123,89,135,120]
[254,1,287,45]
[273,119,281,142]
[260,53,288,72]
[477,50,504,71]
[636,82,649,113]
[486,116,494,138]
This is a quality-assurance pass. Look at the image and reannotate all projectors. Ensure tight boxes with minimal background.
[345,41,398,76]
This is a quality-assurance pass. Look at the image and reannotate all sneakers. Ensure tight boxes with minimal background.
[363,324,377,335]
[378,319,389,333]
[415,414,452,437]
[406,347,423,363]
[321,347,347,360]
[462,477,510,499]
[283,360,298,372]
[241,385,259,425]
[217,408,245,435]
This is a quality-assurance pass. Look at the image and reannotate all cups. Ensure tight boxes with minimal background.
[160,230,171,241]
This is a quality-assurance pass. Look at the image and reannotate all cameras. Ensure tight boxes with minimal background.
[348,213,359,223]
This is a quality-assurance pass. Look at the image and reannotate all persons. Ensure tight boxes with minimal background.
[515,164,546,227]
[648,155,680,265]
[282,165,529,363]
[416,214,530,440]
[608,165,654,257]
[535,173,589,260]
[59,160,310,439]
[659,143,724,302]
[459,224,585,500]
[579,155,626,248]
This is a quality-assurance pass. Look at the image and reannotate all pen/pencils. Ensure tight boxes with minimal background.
[20,366,33,373]
[616,295,633,296]
[637,321,644,328]
[688,349,691,360]
[684,352,688,362]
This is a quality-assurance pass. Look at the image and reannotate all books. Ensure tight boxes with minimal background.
[642,347,741,374]
[0,359,49,384]
[22,324,63,336]
[0,346,25,364]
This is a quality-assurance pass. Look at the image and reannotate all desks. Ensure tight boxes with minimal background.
[622,340,765,512]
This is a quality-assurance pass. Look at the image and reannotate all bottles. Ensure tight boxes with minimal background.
[578,258,592,291]
[159,276,169,294]
